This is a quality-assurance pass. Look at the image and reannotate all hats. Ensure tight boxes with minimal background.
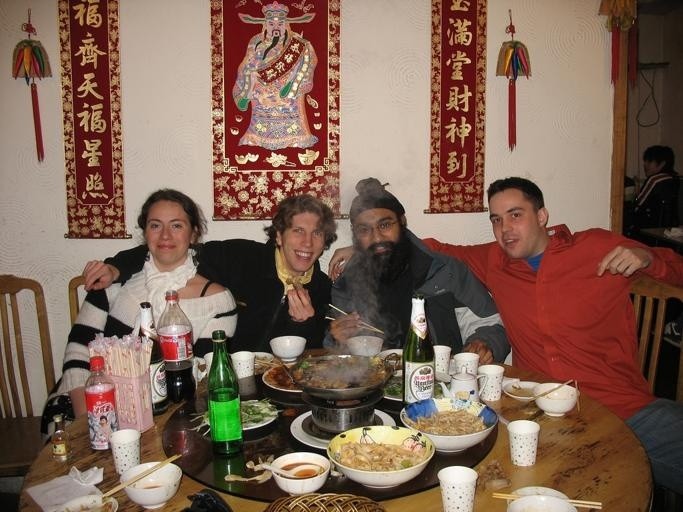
[350,177,405,223]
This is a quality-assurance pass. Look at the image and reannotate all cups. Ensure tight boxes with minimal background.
[203,351,214,376]
[230,350,255,379]
[437,465,478,512]
[108,429,141,476]
[477,365,505,402]
[507,420,540,467]
[433,345,452,379]
[454,352,479,378]
[237,377,256,396]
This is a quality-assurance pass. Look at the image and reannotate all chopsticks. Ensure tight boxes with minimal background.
[492,492,602,510]
[324,303,385,335]
[88,334,153,427]
[527,378,581,412]
[100,454,180,497]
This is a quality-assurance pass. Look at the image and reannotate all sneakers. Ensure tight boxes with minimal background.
[650,320,682,347]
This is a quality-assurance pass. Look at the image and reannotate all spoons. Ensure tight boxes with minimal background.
[260,464,321,478]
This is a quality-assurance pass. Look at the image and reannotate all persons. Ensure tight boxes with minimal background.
[633,146,683,214]
[327,177,682,500]
[322,178,511,366]
[82,194,337,355]
[232,6,319,148]
[39,187,238,448]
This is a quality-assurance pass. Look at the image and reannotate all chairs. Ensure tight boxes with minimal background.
[1,275,58,478]
[628,278,683,402]
[68,276,89,323]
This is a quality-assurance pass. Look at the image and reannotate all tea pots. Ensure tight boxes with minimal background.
[438,367,487,403]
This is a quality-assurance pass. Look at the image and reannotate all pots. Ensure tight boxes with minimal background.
[282,352,400,402]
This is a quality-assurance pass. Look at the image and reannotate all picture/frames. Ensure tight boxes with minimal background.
[207,0,350,220]
[423,1,490,214]
[58,0,134,240]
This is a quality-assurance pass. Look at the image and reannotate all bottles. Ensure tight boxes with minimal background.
[400,292,437,407]
[137,300,170,417]
[156,289,198,404]
[51,413,73,464]
[84,354,120,452]
[207,329,245,459]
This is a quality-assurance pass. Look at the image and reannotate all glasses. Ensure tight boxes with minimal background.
[350,220,403,237]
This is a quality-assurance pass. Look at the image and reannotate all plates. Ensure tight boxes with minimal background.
[204,406,276,433]
[374,348,403,361]
[290,408,396,450]
[251,352,275,369]
[57,494,119,512]
[503,381,539,403]
[301,413,384,444]
[506,486,569,504]
[383,370,443,402]
[260,367,304,394]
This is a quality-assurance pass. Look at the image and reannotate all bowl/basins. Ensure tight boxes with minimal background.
[347,335,383,356]
[506,495,577,512]
[399,397,498,453]
[120,461,182,509]
[270,336,307,362]
[271,452,330,496]
[533,383,580,417]
[326,425,435,489]
